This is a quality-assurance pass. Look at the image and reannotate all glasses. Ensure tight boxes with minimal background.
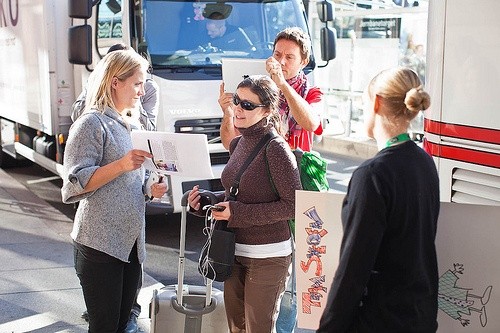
[233,95,265,110]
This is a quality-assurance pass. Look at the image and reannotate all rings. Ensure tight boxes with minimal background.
[272,63,275,69]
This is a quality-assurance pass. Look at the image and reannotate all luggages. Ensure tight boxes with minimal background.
[149,190,228,333]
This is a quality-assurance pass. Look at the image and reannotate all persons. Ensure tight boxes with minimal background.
[202,11,257,50]
[71,45,160,333]
[317,66,440,333]
[216,27,327,333]
[60,51,168,333]
[187,75,303,333]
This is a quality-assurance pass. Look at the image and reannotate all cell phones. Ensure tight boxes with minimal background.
[210,206,226,212]
[143,170,163,201]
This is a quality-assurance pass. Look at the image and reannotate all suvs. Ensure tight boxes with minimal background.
[307,1,429,106]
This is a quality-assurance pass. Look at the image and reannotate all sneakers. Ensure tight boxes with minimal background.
[80,311,88,318]
[125,313,139,333]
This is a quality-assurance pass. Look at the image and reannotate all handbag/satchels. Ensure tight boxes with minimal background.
[197,228,236,282]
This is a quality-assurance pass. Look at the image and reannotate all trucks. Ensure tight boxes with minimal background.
[0,0,337,219]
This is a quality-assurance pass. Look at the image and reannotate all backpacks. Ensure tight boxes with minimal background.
[265,136,329,240]
